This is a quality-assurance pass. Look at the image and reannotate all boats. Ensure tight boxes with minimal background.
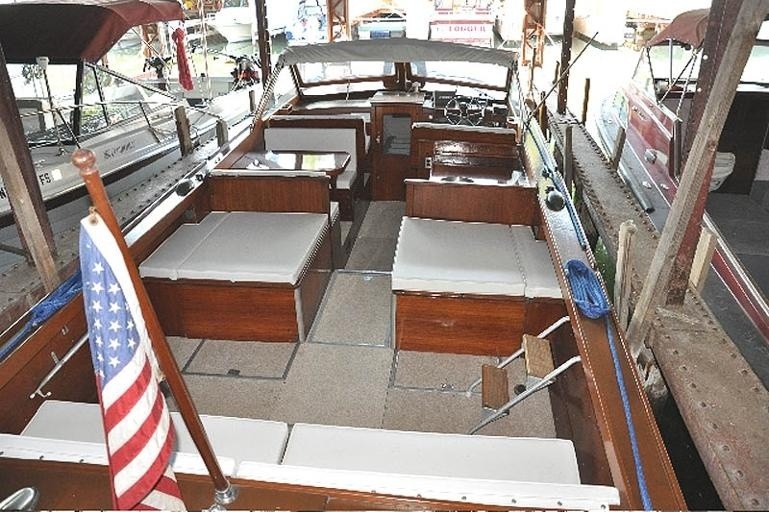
[208,7,252,43]
[286,0,406,40]
[0,0,294,216]
[596,10,767,398]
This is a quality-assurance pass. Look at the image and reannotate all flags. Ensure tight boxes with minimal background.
[76,210,194,510]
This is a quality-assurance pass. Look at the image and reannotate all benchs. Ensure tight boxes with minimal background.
[138,113,565,356]
[1,399,620,512]
